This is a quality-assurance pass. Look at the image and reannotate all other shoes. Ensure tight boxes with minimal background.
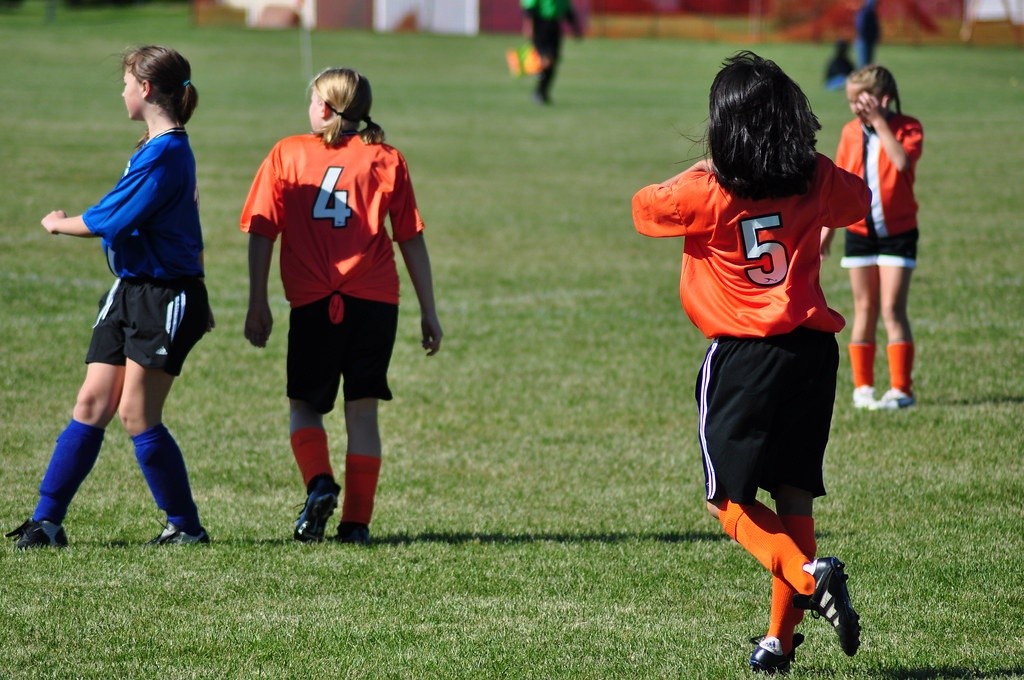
[854,388,883,409]
[330,522,370,544]
[295,483,341,541]
[882,390,915,412]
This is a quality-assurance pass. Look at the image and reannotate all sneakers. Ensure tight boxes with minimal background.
[750,632,804,673]
[5,518,67,548]
[793,558,862,656]
[147,522,209,545]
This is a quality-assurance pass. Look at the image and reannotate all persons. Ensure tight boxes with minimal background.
[239,65,442,546]
[3,42,220,547]
[632,50,875,672]
[520,0,586,104]
[855,0,882,68]
[821,39,859,91]
[816,63,925,409]
